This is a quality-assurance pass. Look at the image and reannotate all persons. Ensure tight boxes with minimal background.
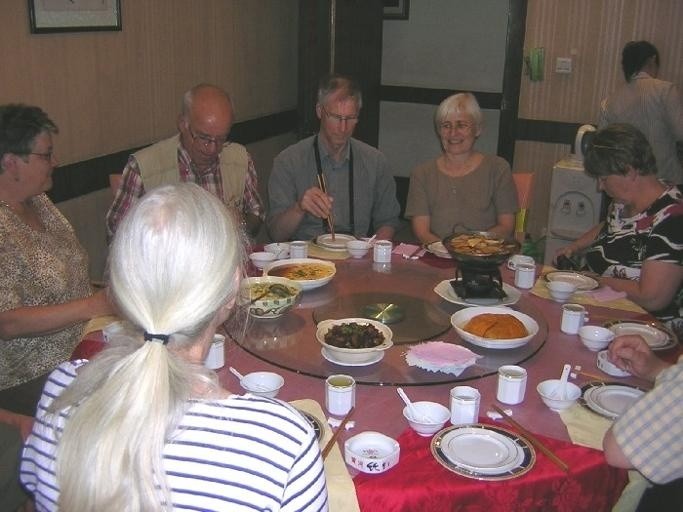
[602,333,683,512]
[556,121,683,349]
[18,182,331,512]
[606,39,683,194]
[0,103,117,421]
[265,75,403,246]
[403,91,520,251]
[105,83,266,251]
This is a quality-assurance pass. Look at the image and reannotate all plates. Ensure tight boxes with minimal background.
[602,317,678,351]
[542,269,601,293]
[429,423,536,481]
[301,410,325,442]
[576,380,649,420]
[422,239,451,259]
[311,233,360,251]
[432,276,522,308]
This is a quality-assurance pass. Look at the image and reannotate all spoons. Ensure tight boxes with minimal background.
[394,386,432,424]
[228,367,268,392]
[550,364,571,402]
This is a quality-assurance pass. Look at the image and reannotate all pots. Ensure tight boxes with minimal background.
[314,317,393,361]
[442,223,521,267]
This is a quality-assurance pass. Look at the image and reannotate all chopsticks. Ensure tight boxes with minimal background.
[316,173,335,241]
[492,403,568,471]
[571,370,606,382]
[321,406,355,459]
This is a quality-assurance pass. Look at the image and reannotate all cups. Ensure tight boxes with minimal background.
[496,364,528,405]
[248,240,309,270]
[372,241,392,263]
[447,385,481,424]
[507,254,534,270]
[558,303,589,335]
[202,333,226,369]
[324,374,356,416]
[511,263,536,289]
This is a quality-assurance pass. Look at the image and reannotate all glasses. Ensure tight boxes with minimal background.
[186,125,232,148]
[320,107,360,124]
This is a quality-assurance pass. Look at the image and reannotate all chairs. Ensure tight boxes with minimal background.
[510,172,533,242]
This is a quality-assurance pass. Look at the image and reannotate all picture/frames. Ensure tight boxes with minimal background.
[383,0,409,20]
[27,0,123,34]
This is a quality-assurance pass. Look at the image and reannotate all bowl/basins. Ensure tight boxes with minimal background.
[450,306,539,349]
[401,400,451,437]
[239,371,284,399]
[544,281,576,303]
[537,379,582,412]
[346,240,371,258]
[236,276,303,318]
[319,346,384,367]
[578,325,616,352]
[263,258,336,291]
